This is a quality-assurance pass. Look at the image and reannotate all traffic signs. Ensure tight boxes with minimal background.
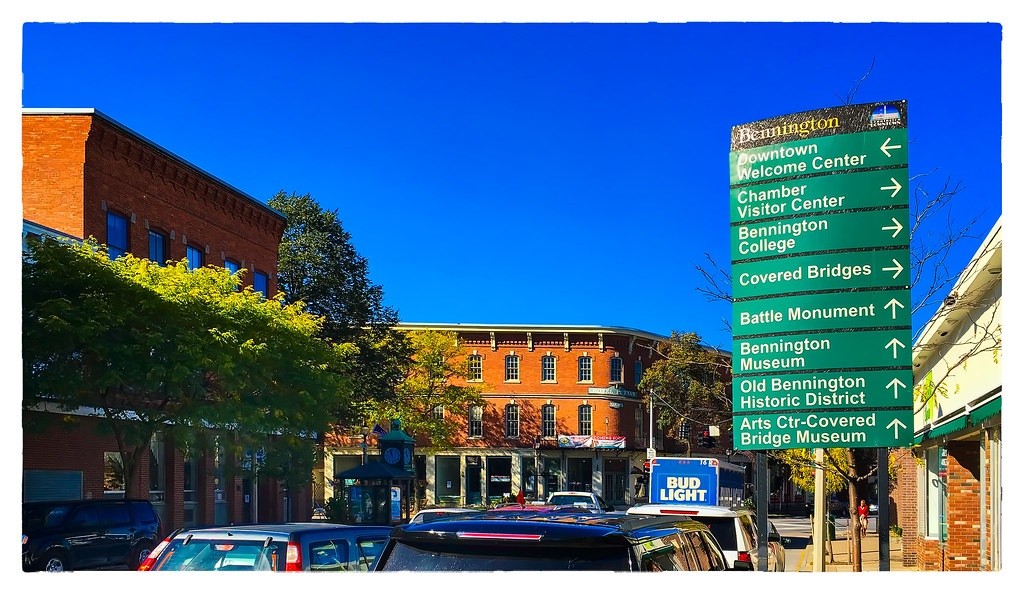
[728,128,913,450]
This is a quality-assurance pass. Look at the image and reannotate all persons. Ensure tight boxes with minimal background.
[858,500,869,538]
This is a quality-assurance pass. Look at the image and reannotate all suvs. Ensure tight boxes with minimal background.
[626,504,780,570]
[368,511,754,571]
[544,492,616,511]
[409,508,484,523]
[486,503,590,513]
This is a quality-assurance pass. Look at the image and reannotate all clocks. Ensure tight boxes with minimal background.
[384,447,401,464]
[404,448,410,464]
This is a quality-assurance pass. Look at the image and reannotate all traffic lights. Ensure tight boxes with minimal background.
[697,428,717,448]
[644,462,650,473]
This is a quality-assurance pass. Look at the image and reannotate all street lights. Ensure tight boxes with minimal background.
[361,418,369,465]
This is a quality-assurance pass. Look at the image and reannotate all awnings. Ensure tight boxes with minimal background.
[968,392,1001,425]
[910,429,929,445]
[930,412,966,438]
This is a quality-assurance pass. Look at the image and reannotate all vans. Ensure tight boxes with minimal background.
[136,522,393,571]
[22,499,163,572]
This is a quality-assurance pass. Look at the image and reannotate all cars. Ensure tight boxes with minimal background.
[869,505,879,515]
[805,498,848,518]
[767,520,791,572]
